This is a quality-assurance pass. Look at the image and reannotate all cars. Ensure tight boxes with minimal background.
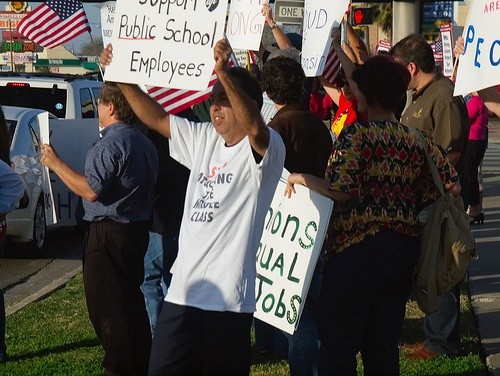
[0,105,57,255]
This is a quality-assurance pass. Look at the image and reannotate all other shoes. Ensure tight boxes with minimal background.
[401,342,436,359]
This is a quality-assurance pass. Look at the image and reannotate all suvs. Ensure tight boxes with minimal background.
[0,70,106,120]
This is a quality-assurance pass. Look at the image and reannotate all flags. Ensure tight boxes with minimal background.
[144,56,235,116]
[15,1,92,50]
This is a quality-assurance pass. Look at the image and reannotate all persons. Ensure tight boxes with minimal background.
[100,44,285,376]
[260,3,500,376]
[40,81,161,376]
[0,103,31,363]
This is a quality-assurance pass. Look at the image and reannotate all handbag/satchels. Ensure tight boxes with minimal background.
[410,127,476,314]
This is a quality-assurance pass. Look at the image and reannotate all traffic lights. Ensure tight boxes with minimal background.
[352,7,374,25]
